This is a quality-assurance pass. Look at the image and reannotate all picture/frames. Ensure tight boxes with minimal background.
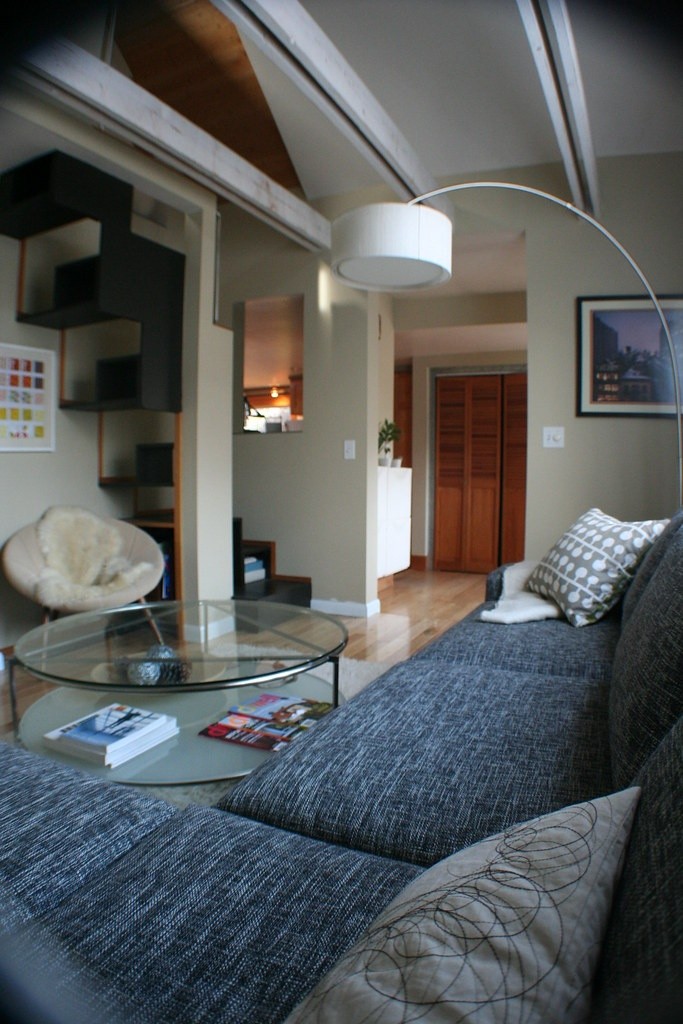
[573,294,683,419]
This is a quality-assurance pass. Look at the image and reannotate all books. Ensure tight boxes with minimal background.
[42,702,182,769]
[196,693,335,749]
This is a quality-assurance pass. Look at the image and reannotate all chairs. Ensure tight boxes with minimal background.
[0,503,165,661]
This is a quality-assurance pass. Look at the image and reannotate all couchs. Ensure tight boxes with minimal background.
[1,511,683,1024]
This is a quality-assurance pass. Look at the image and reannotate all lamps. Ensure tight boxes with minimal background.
[329,181,683,520]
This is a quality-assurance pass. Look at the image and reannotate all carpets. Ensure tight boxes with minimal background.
[0,642,394,808]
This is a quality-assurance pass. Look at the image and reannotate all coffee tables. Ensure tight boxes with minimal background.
[8,599,350,785]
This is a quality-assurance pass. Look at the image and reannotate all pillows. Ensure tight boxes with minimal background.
[521,506,672,628]
[284,785,643,1024]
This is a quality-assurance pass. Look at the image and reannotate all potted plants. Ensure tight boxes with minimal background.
[377,418,401,467]
